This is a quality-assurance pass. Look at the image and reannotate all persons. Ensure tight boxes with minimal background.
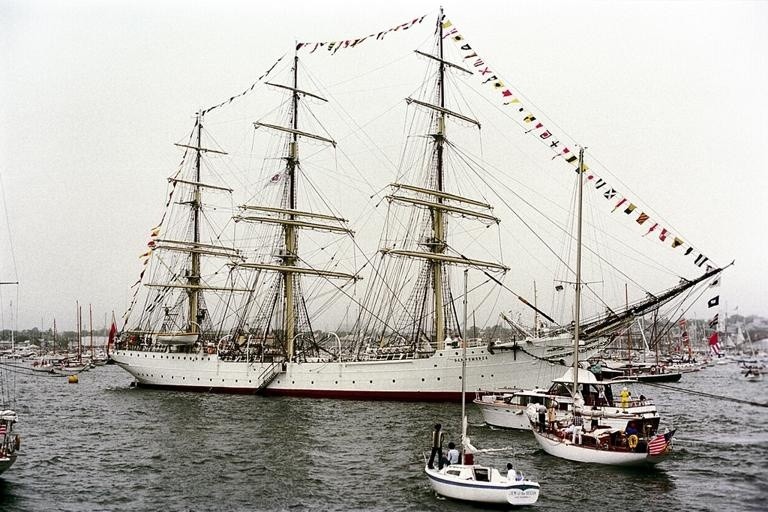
[442,442,460,466]
[638,395,646,406]
[620,387,629,413]
[428,424,444,470]
[599,387,604,406]
[627,422,639,448]
[535,400,586,446]
[628,392,637,407]
[506,463,517,482]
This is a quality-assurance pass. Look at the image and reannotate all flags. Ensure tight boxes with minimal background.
[648,428,677,455]
[106,322,116,356]
[119,117,210,324]
[295,9,429,55]
[197,54,294,115]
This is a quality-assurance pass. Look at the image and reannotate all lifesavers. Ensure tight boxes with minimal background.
[651,367,655,372]
[628,434,638,448]
[16,435,20,450]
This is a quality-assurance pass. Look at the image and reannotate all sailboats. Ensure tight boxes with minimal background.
[110,10,719,405]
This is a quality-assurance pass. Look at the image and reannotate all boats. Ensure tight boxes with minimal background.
[424,367,677,507]
[0,411,20,471]
[30,350,107,375]
[597,347,714,381]
